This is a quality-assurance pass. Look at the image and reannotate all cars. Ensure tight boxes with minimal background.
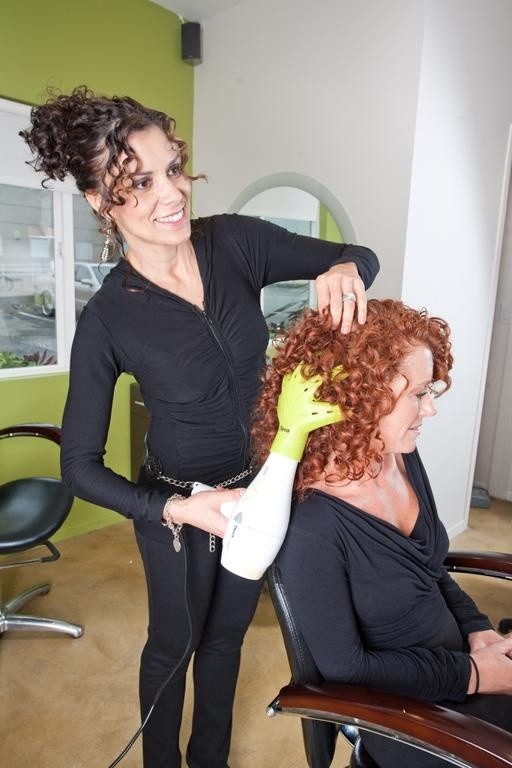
[31,262,122,320]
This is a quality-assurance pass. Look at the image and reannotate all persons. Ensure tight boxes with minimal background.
[19,85,380,768]
[251,299,512,768]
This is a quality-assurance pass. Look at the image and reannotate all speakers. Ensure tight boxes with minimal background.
[181,21,200,65]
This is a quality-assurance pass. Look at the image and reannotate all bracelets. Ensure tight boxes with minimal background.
[163,493,187,552]
[469,655,479,694]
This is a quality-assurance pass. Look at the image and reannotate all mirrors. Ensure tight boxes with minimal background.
[218,168,355,345]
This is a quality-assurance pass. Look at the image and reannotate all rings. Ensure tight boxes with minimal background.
[342,292,356,302]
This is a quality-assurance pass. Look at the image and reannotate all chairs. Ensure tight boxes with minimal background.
[0,419,90,642]
[250,514,511,766]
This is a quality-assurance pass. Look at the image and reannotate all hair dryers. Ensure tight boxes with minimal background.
[192,359,352,582]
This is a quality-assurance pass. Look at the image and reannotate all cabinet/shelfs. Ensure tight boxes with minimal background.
[126,380,157,485]
